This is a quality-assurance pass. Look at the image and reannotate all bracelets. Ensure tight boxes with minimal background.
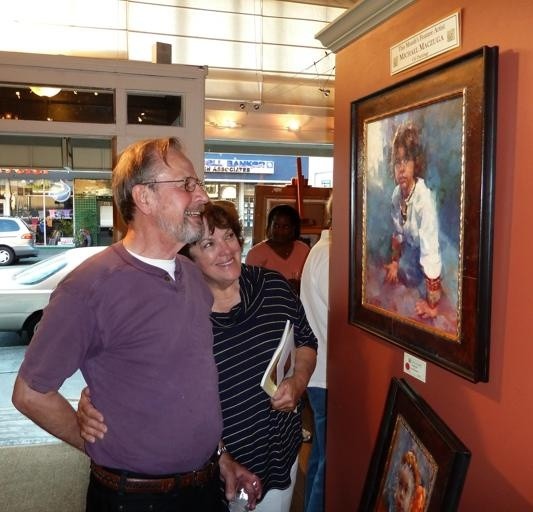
[217,444,229,460]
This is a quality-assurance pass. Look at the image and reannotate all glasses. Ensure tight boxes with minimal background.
[141,176,205,193]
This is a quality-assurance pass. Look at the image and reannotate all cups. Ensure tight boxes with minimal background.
[227,473,262,511]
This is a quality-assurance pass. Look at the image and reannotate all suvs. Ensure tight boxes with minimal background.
[0,217,38,265]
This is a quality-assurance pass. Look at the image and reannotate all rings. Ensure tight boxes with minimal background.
[284,408,290,412]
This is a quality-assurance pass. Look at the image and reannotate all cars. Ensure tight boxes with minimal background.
[1,246,110,346]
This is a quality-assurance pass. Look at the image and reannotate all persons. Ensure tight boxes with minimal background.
[298,190,333,512]
[385,122,446,318]
[245,204,312,443]
[390,448,427,512]
[179,198,320,512]
[12,135,264,512]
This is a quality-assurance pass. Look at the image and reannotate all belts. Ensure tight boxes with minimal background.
[90,458,218,496]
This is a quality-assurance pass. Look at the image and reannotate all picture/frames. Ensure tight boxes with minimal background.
[346,44,499,385]
[355,375,471,512]
